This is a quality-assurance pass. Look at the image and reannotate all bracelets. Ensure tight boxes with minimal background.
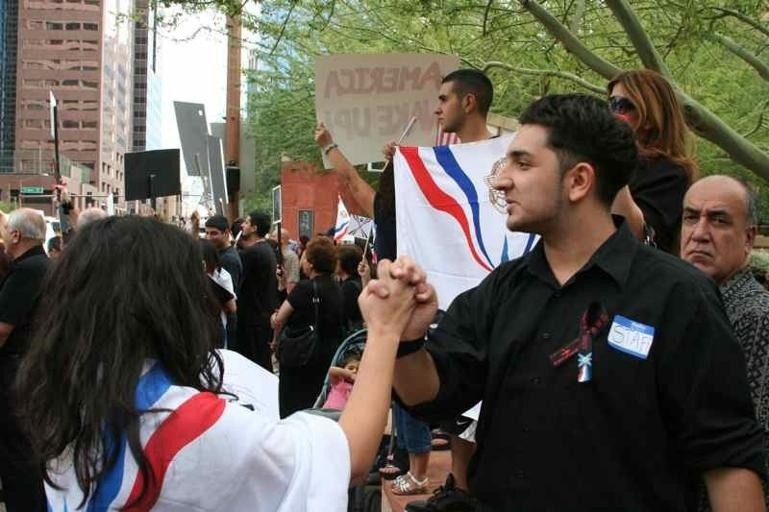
[323,143,338,153]
[396,332,427,358]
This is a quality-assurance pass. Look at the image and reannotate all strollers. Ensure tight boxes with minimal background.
[309,327,388,512]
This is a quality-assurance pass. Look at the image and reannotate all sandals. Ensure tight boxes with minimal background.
[392,475,433,496]
[389,471,413,488]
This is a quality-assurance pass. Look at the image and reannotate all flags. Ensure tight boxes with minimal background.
[393,129,541,312]
[348,214,371,240]
[332,195,355,245]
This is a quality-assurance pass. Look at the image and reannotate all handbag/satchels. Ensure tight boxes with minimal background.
[275,283,319,369]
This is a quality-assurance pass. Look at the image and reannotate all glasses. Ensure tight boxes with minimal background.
[603,95,635,115]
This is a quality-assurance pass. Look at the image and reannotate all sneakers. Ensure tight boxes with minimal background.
[404,473,474,512]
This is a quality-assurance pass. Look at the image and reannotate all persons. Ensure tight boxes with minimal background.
[269,236,340,420]
[270,228,300,311]
[365,92,769,511]
[190,210,244,307]
[321,355,361,413]
[46,235,62,257]
[605,69,696,259]
[678,171,769,427]
[0,207,58,373]
[198,236,237,350]
[334,243,363,333]
[312,120,432,495]
[238,209,277,374]
[383,68,500,511]
[54,183,107,232]
[1,214,417,512]
[357,262,408,480]
